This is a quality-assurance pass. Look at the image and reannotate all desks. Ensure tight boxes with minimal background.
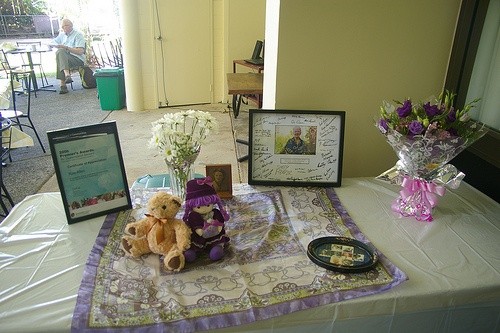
[0,78,11,110]
[0,177,500,333]
[11,47,56,96]
[233,60,264,119]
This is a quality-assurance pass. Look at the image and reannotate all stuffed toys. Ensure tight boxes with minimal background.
[121,191,192,273]
[182,176,230,262]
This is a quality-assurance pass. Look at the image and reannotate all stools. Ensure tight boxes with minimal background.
[227,73,263,162]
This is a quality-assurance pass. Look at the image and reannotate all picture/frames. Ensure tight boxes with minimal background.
[46,120,133,225]
[247,109,347,187]
[206,164,233,198]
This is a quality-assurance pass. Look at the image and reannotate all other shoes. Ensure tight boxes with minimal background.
[64,75,74,84]
[59,85,68,94]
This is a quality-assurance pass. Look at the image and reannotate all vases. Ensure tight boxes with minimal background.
[165,160,191,200]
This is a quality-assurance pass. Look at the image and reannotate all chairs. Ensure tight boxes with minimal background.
[0,41,86,218]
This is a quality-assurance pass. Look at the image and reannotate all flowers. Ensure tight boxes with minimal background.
[374,89,484,176]
[149,110,216,199]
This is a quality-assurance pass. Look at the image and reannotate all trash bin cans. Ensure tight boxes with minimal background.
[92,67,126,111]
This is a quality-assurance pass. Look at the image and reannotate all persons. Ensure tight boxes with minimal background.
[49,18,86,94]
[281,126,307,155]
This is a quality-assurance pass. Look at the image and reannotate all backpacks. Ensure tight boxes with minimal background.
[79,63,98,89]
